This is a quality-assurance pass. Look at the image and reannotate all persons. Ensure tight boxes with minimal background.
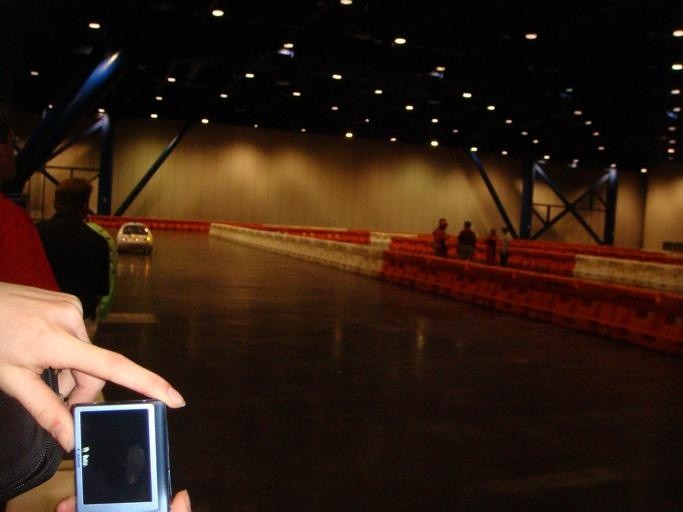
[432,218,446,236]
[0,116,65,291]
[498,227,509,267]
[433,221,449,256]
[455,220,477,260]
[34,176,119,346]
[484,228,497,262]
[0,277,195,512]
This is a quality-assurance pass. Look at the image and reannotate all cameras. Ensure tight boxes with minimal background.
[73,400,172,512]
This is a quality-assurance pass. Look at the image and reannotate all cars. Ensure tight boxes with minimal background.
[113,218,158,260]
